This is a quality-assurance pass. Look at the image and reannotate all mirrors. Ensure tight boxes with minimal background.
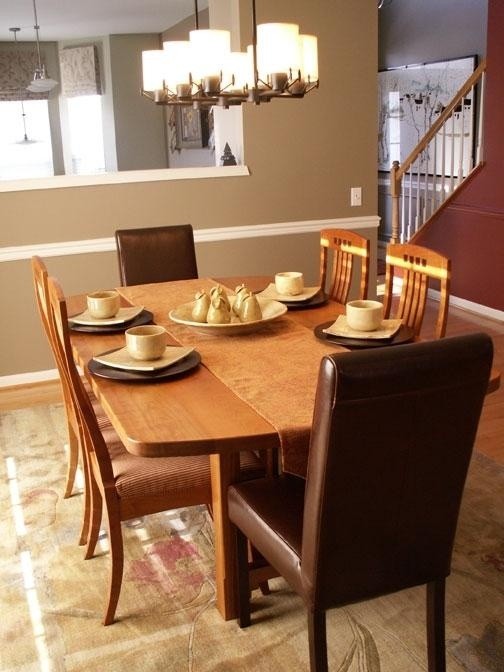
[0,0,251,191]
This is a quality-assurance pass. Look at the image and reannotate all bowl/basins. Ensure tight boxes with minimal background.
[86,291,121,319]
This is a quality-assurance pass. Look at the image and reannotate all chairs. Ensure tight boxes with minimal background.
[27,253,125,550]
[113,223,200,290]
[252,236,452,489]
[211,330,497,672]
[44,274,276,633]
[309,227,372,308]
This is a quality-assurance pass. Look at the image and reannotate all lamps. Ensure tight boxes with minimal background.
[188,0,323,115]
[6,1,59,147]
[133,0,255,110]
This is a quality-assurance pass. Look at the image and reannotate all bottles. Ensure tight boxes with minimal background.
[194,283,261,323]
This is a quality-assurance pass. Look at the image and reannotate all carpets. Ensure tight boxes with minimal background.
[0,398,504,671]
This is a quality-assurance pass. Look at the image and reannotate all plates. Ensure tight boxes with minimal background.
[84,348,205,383]
[312,320,416,350]
[254,281,324,303]
[321,314,406,339]
[168,297,288,337]
[285,294,329,308]
[67,305,146,326]
[65,311,154,334]
[92,343,197,372]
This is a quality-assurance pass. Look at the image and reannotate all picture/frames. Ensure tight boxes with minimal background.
[170,101,210,151]
[374,53,480,181]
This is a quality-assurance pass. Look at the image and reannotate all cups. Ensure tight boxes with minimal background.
[273,271,304,294]
[344,299,383,332]
[123,324,167,360]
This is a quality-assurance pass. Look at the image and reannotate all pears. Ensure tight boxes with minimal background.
[191,283,262,324]
